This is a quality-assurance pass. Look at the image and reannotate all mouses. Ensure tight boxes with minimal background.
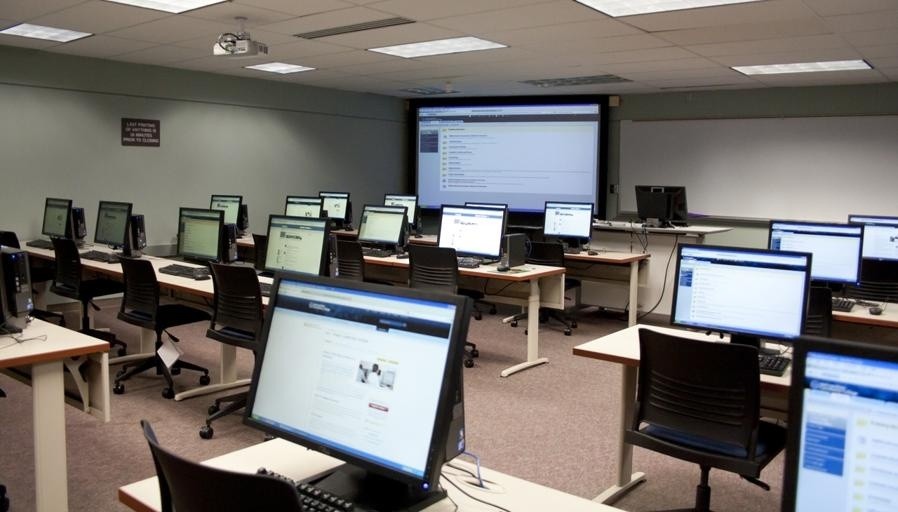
[869,308,882,314]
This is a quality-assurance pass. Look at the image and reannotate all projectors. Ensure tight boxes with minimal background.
[212,40,268,57]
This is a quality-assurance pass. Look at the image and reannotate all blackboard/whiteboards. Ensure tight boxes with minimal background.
[617,113,898,226]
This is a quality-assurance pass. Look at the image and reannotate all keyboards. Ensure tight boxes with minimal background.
[256,467,363,512]
[26,239,57,250]
[256,280,273,296]
[758,353,791,375]
[832,299,854,312]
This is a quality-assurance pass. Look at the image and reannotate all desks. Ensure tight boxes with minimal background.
[0,314,112,512]
[238,237,566,377]
[118,435,632,512]
[572,323,795,504]
[564,250,651,326]
[14,236,275,401]
[592,218,733,244]
[831,296,898,327]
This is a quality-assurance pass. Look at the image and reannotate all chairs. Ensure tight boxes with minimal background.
[406,243,478,368]
[49,234,126,356]
[251,234,275,277]
[198,259,266,437]
[2,231,66,327]
[113,251,211,398]
[336,240,366,282]
[624,328,787,511]
[140,419,306,512]
[511,241,578,335]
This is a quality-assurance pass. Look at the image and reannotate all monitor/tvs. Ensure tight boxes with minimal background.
[357,204,408,249]
[93,197,133,247]
[177,206,224,262]
[439,200,508,260]
[847,214,898,261]
[767,220,865,288]
[42,198,74,237]
[670,244,813,355]
[209,195,243,229]
[544,200,594,244]
[265,214,331,277]
[285,195,325,218]
[383,195,421,227]
[781,335,898,512]
[243,270,474,512]
[319,189,353,226]
[635,185,687,226]
